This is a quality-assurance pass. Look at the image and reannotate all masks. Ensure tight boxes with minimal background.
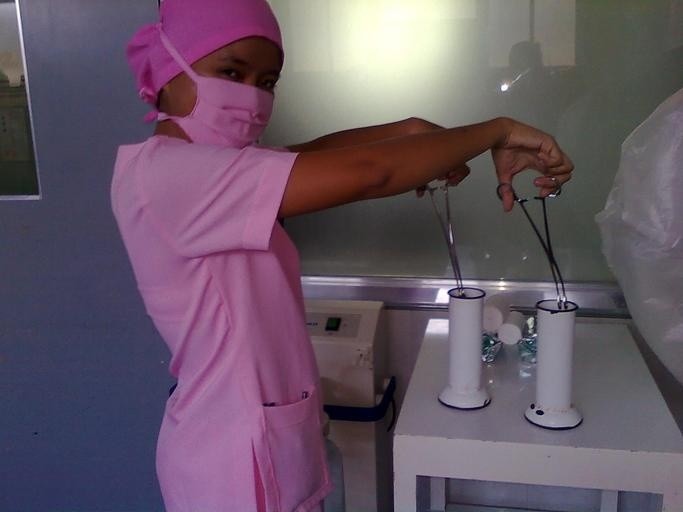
[157,23,275,148]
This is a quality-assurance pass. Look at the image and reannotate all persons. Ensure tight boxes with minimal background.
[110,1,576,512]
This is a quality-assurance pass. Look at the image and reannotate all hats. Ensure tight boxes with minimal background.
[126,1,284,94]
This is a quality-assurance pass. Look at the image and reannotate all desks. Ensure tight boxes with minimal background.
[391,316,682,511]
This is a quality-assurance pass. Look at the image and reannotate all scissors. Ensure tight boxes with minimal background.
[415,181,466,297]
[496,177,568,313]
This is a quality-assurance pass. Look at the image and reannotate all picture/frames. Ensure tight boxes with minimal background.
[0,0,43,204]
